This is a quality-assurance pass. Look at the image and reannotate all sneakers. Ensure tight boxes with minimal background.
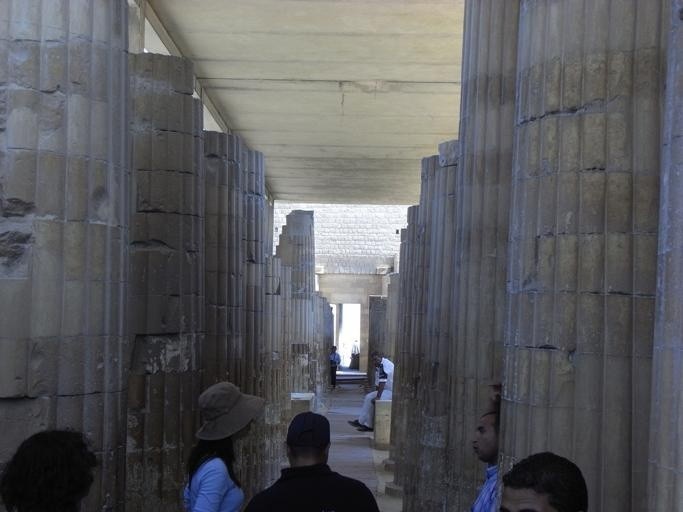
[348,419,374,432]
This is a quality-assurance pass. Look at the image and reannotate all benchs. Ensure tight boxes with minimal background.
[375,400,392,447]
[291,392,314,415]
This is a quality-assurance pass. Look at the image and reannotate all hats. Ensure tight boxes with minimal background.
[194,381,267,442]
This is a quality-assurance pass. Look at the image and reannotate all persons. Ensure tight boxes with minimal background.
[180,380,266,511]
[0,428,99,511]
[329,346,340,387]
[498,450,589,511]
[241,411,381,511]
[350,338,360,369]
[468,381,502,511]
[346,350,394,431]
